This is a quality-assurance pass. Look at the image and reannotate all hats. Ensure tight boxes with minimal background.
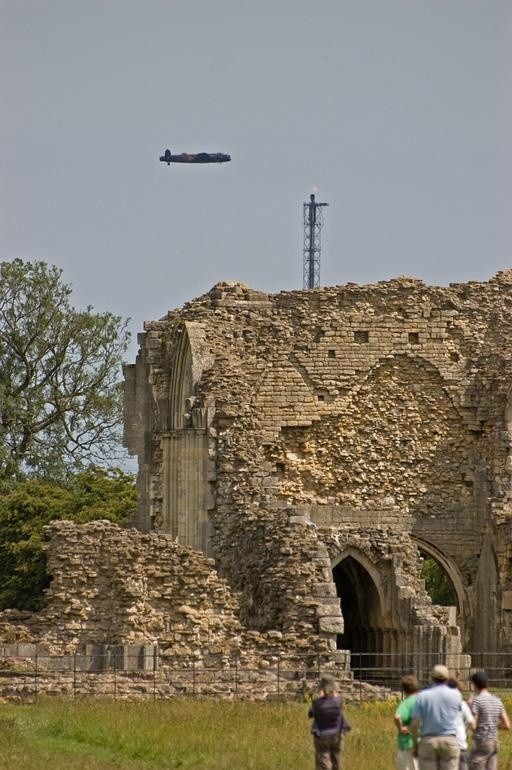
[469,672,487,685]
[432,665,448,680]
[318,675,338,691]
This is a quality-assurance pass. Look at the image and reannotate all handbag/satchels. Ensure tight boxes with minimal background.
[315,735,343,753]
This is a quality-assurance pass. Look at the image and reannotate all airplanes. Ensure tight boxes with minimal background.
[160,150,231,165]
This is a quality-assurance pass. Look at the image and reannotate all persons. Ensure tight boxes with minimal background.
[392,662,510,770]
[307,674,351,770]
